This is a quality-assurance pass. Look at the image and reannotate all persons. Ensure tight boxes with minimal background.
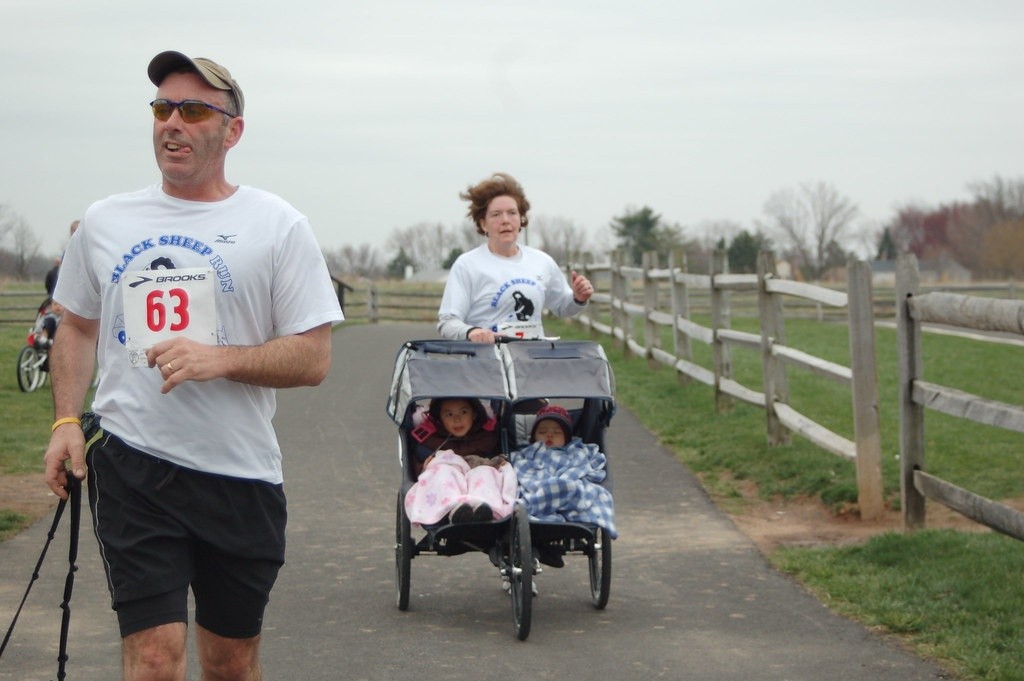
[530,406,573,450]
[32,52,345,681]
[410,397,506,530]
[437,173,594,598]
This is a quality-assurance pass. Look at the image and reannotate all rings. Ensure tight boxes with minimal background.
[167,363,175,374]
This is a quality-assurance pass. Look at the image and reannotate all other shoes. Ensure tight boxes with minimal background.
[448,501,474,524]
[500,557,544,576]
[503,579,539,596]
[473,501,492,521]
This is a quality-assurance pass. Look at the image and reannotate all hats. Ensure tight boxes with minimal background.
[531,406,574,445]
[147,50,245,117]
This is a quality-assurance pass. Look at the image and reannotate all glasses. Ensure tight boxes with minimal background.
[150,99,236,123]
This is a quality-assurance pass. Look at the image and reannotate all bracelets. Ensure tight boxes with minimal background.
[573,294,588,306]
[52,417,82,432]
[466,327,483,341]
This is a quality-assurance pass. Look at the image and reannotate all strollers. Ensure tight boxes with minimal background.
[394,336,614,640]
[16,294,101,394]
